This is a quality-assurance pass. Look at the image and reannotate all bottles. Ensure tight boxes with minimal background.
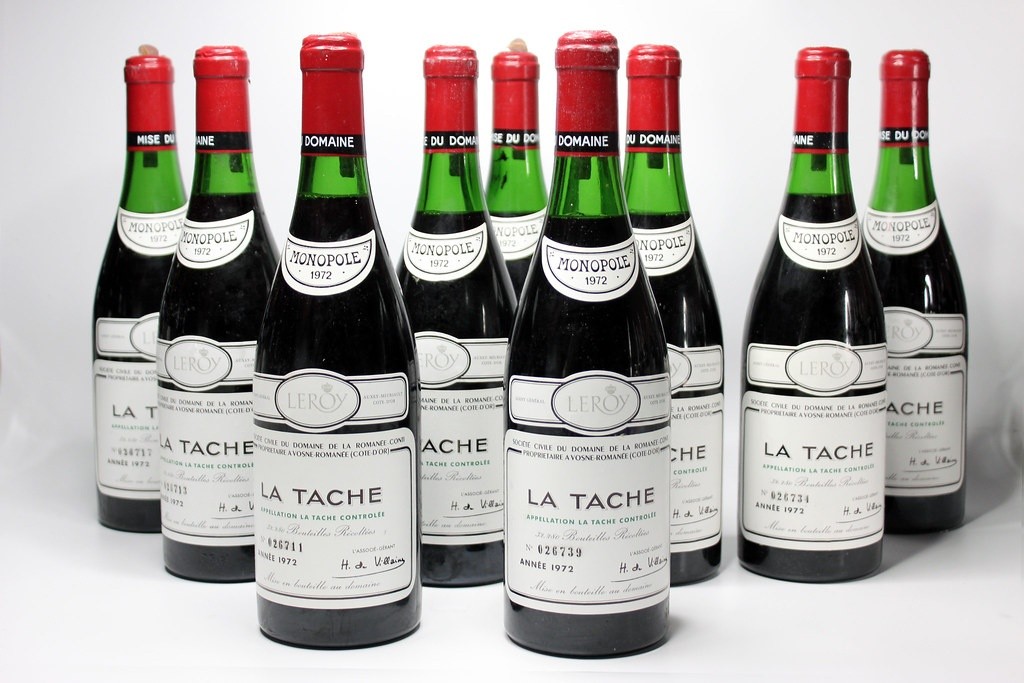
[732,45,890,584]
[854,45,969,539]
[396,44,519,590]
[496,28,672,659]
[156,45,280,585]
[248,30,423,651]
[620,44,726,589]
[90,52,191,535]
[481,51,552,308]
